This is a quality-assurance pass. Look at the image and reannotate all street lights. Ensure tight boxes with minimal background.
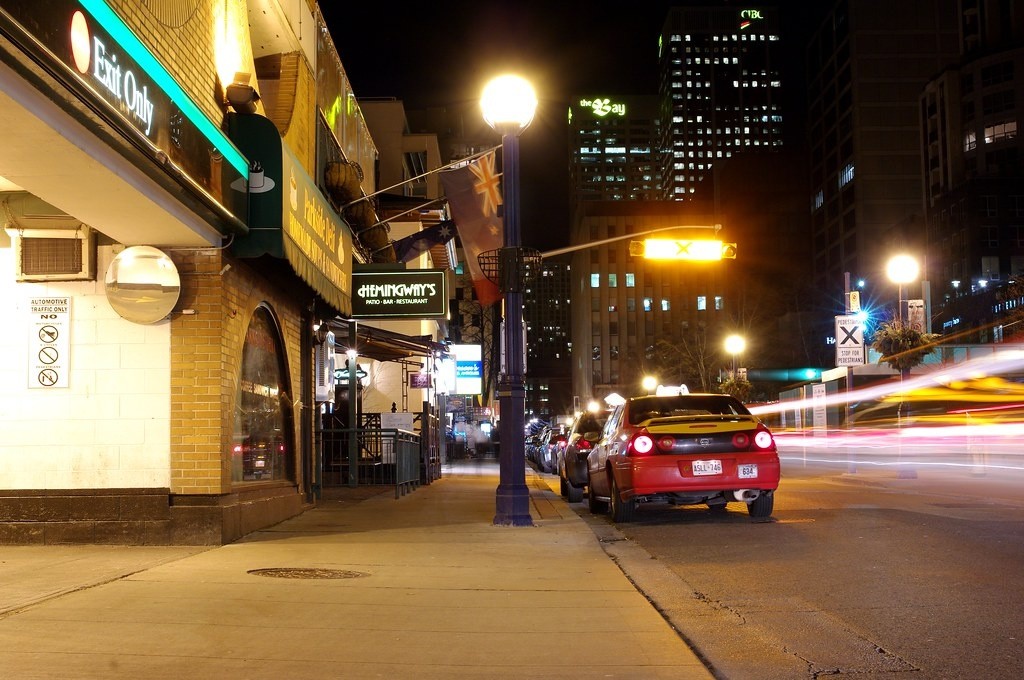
[479,74,538,528]
[724,335,745,382]
[884,252,920,384]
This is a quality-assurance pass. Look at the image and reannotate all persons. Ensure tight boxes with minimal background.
[491,421,501,462]
[472,423,487,462]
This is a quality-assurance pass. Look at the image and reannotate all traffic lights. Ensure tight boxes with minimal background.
[628,237,738,262]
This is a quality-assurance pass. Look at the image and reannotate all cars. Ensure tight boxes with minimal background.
[582,383,782,524]
[525,408,616,502]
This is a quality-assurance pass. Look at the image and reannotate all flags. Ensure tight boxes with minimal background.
[437,151,504,307]
[391,219,458,263]
